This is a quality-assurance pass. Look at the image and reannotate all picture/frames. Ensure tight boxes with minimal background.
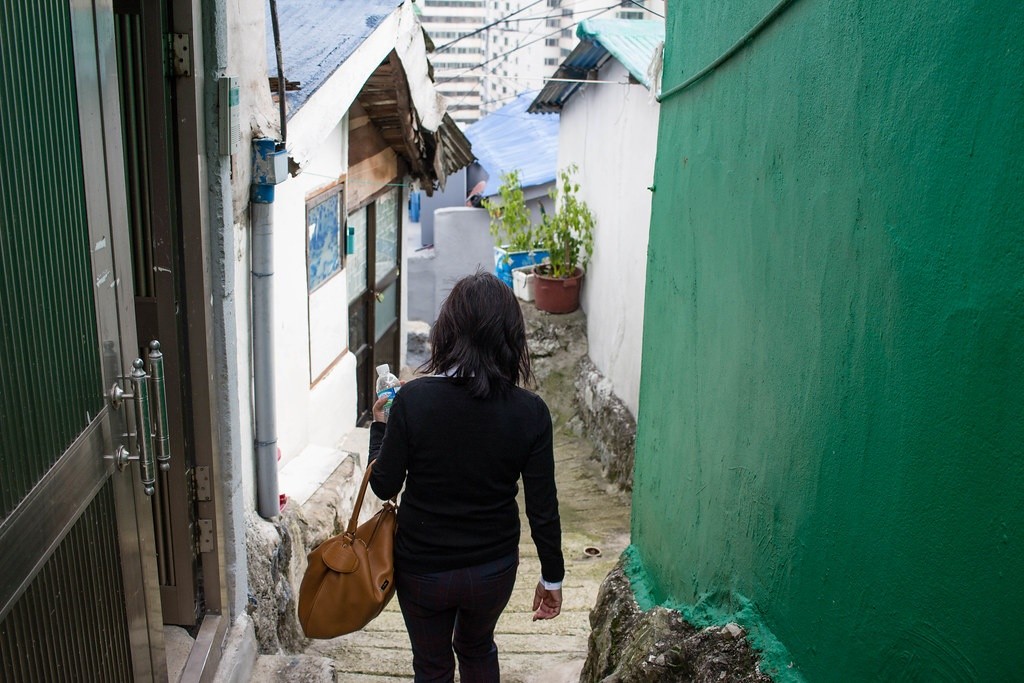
[306,184,343,293]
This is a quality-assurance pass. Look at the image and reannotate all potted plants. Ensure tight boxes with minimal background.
[533,165,594,312]
[481,171,553,285]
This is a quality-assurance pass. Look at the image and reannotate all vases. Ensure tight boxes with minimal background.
[512,264,536,302]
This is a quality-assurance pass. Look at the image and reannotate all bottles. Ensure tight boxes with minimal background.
[376,362,402,418]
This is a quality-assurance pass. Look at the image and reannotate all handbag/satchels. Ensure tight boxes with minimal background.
[296,459,399,641]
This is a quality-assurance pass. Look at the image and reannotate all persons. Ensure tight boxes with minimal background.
[366,272,565,683]
